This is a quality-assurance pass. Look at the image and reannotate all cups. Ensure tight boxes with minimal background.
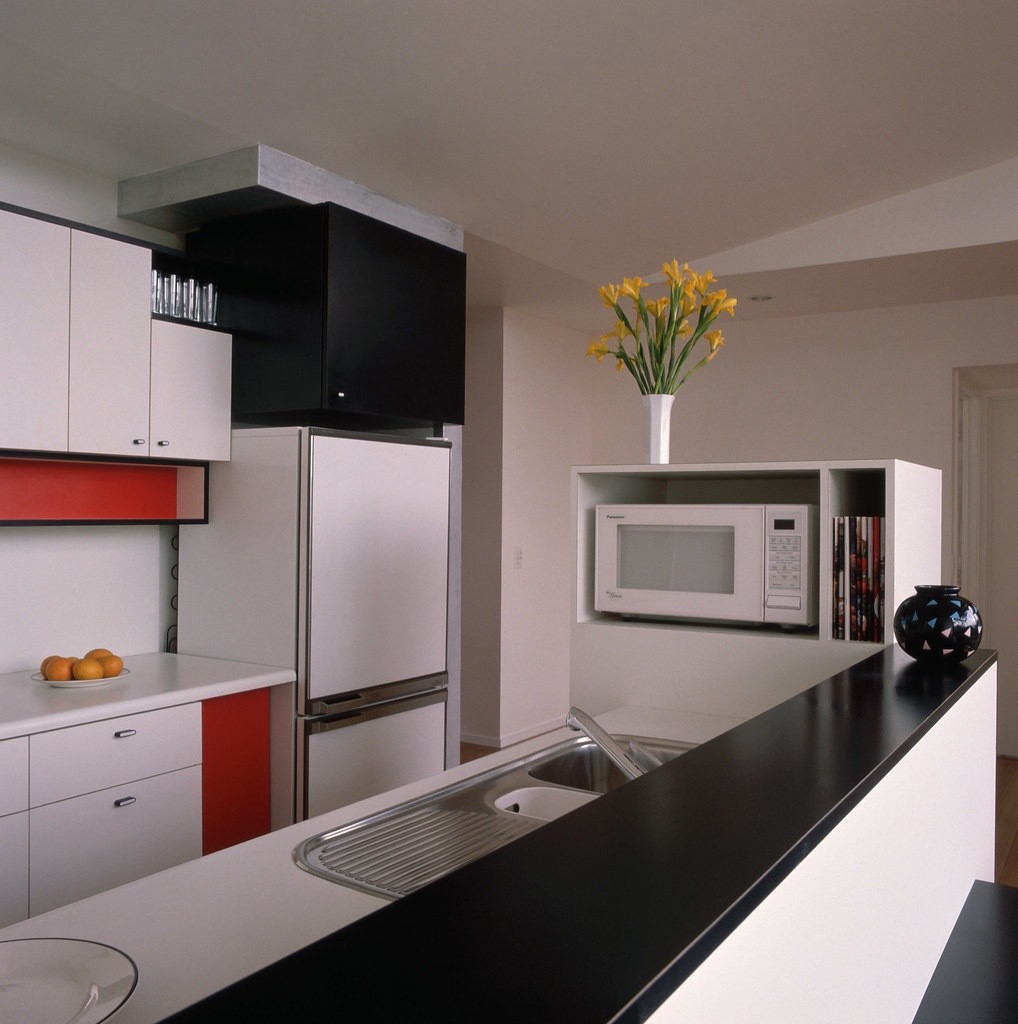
[152,269,218,325]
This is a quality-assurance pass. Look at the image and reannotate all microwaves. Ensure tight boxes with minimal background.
[593,500,817,633]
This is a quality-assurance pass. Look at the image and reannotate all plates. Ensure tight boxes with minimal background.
[1,937,139,1024]
[28,669,131,690]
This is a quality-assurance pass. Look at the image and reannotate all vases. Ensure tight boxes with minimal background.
[641,393,676,466]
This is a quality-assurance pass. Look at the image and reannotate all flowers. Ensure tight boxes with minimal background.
[585,258,738,395]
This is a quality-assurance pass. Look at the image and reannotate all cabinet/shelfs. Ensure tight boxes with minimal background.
[182,200,468,426]
[27,700,203,919]
[0,201,233,464]
[0,735,30,928]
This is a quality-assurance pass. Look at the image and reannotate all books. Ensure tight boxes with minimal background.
[833,515,884,644]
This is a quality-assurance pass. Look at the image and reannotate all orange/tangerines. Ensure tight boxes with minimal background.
[40,648,122,682]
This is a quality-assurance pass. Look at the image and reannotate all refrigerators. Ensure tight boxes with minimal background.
[178,430,450,832]
